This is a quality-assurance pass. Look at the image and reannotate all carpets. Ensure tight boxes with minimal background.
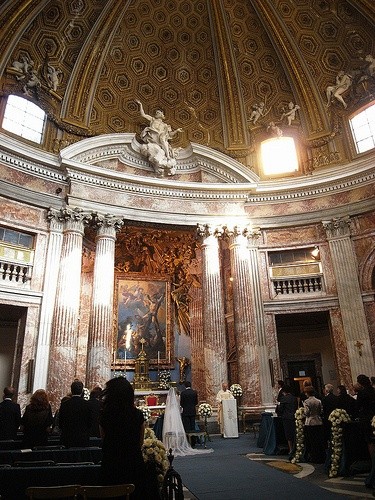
[246,453,375,500]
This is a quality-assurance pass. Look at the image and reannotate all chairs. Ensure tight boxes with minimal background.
[26,485,81,500]
[143,393,160,417]
[79,484,135,500]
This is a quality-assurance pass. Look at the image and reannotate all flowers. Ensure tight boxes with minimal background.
[158,370,171,382]
[113,371,126,378]
[230,384,243,397]
[329,409,351,478]
[291,407,306,464]
[137,406,168,486]
[197,403,213,420]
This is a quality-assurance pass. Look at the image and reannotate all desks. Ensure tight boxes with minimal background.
[256,412,372,475]
[134,390,169,395]
[239,406,277,433]
[0,427,102,500]
[148,406,166,409]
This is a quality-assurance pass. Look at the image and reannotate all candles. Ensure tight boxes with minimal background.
[169,351,171,363]
[142,344,143,350]
[125,351,126,360]
[114,353,115,362]
[158,351,160,364]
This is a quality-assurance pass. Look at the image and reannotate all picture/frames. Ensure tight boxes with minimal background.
[111,272,174,371]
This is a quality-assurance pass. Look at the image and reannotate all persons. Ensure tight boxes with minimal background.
[170,272,190,311]
[134,98,184,160]
[0,375,375,500]
[177,356,188,382]
[249,54,375,138]
